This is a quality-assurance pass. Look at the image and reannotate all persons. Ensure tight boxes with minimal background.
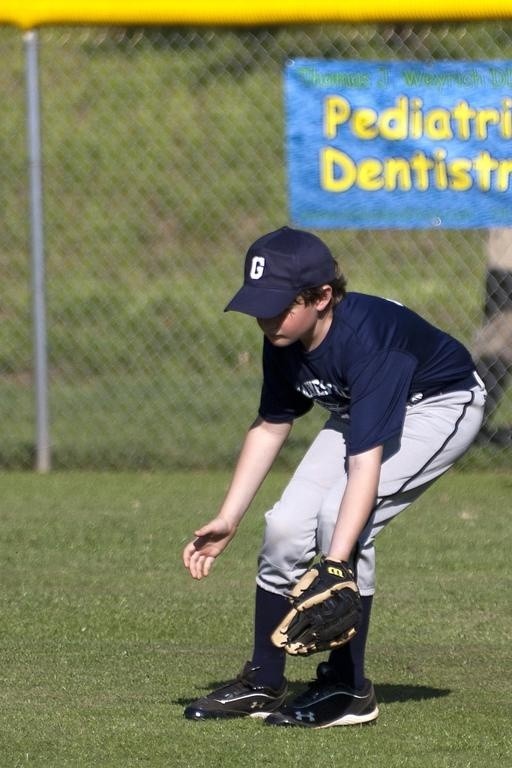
[178,225,490,730]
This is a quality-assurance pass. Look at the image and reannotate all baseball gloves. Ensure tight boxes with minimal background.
[269,556,364,656]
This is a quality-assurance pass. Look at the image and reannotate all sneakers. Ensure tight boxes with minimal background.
[263,660,380,729]
[184,659,289,720]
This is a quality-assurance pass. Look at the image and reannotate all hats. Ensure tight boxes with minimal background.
[223,227,339,319]
